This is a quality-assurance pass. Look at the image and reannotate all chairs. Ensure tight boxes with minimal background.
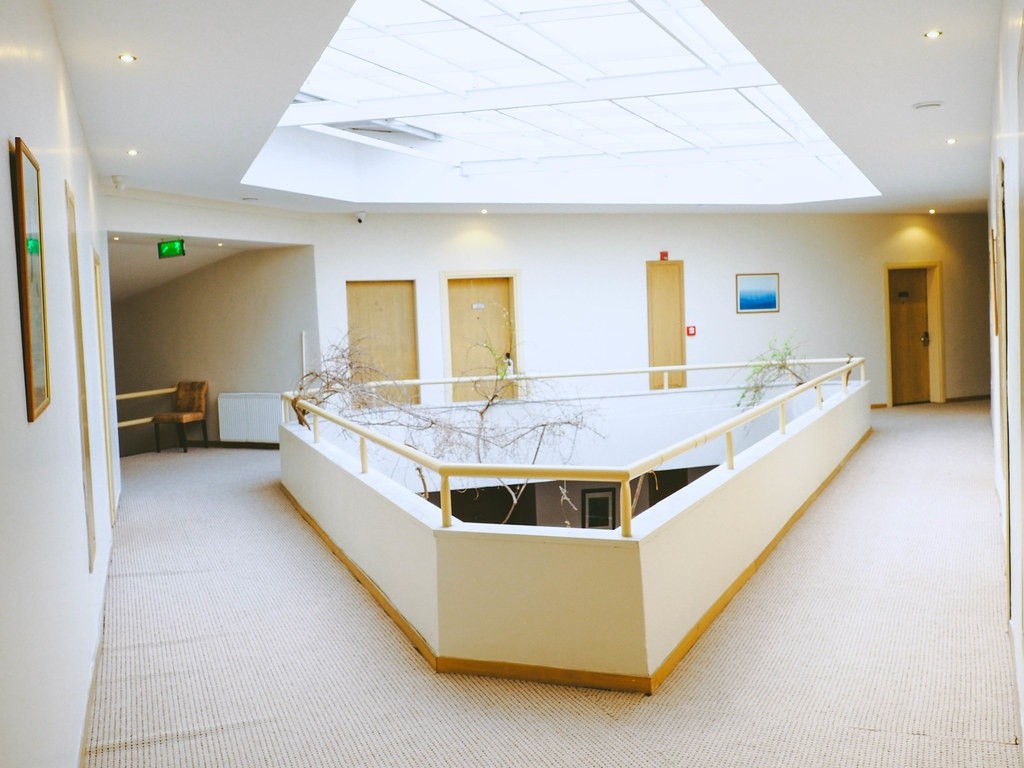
[151,381,210,453]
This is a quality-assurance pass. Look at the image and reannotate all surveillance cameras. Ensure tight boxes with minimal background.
[357,212,366,223]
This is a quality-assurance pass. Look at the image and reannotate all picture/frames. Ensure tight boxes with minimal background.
[735,273,780,314]
[14,137,52,424]
[581,487,616,530]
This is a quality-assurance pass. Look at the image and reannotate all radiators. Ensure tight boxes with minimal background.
[217,393,284,444]
